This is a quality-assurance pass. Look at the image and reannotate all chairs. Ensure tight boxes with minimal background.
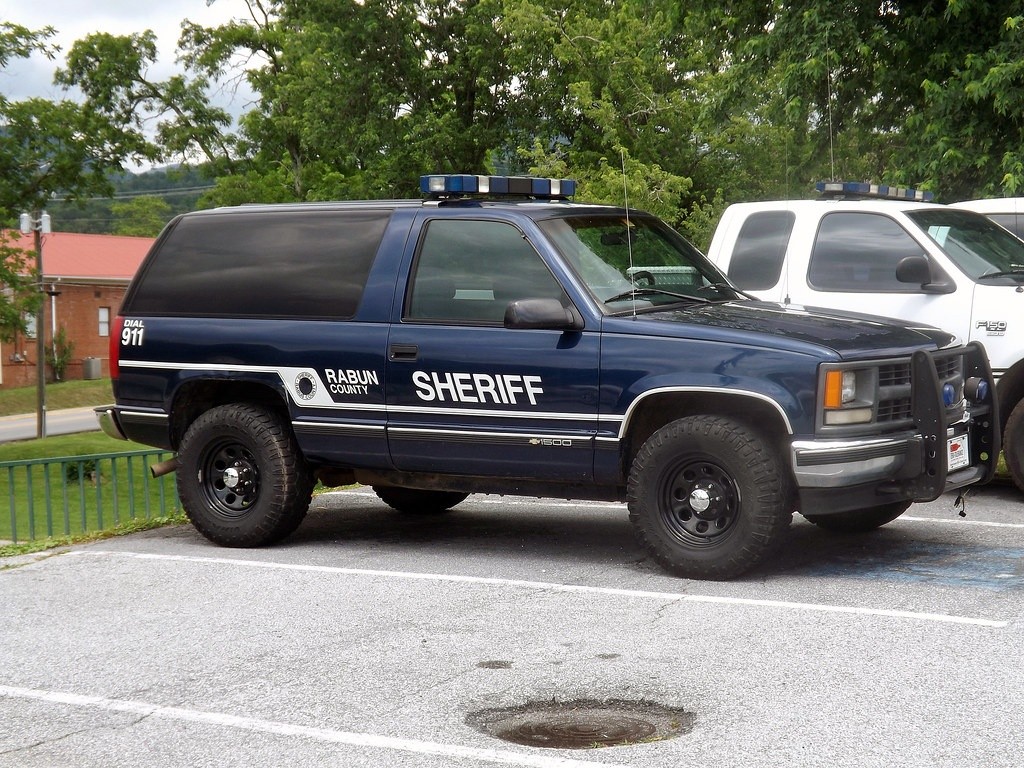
[465,279,529,322]
[419,278,457,317]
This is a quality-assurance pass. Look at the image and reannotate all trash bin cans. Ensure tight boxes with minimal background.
[82,358,103,380]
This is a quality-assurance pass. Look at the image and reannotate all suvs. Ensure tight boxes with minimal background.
[924,196,1024,285]
[94,172,1004,582]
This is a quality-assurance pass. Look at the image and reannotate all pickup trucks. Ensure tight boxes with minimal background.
[625,182,1023,498]
